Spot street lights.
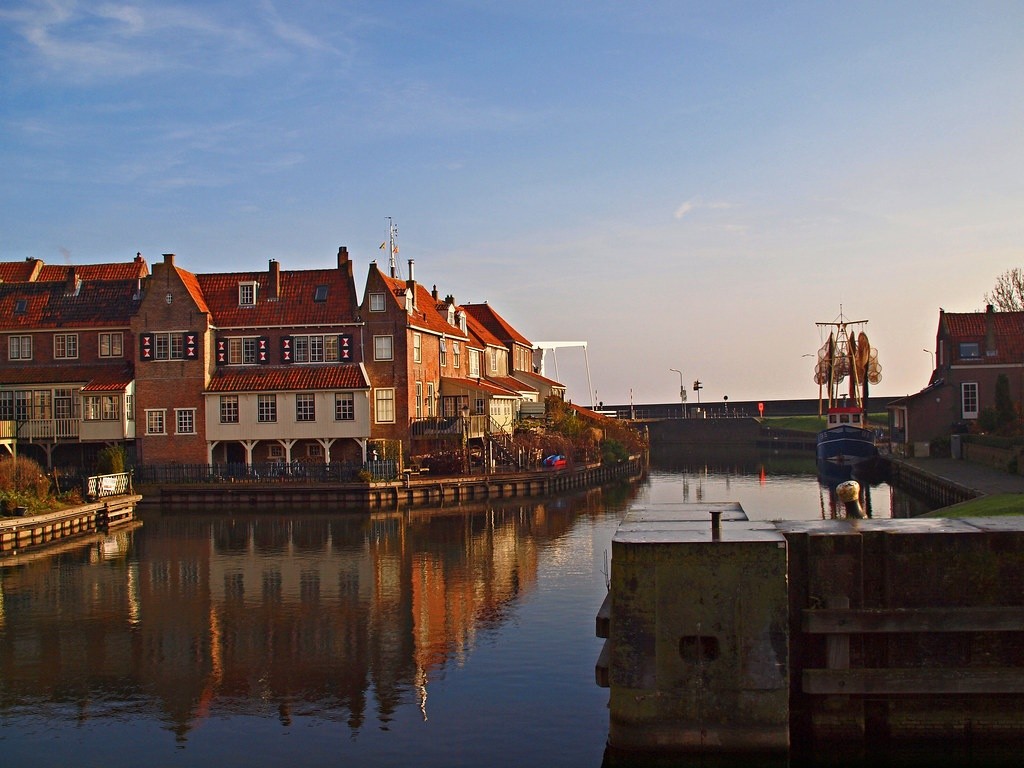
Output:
[924,349,934,370]
[669,369,684,413]
[461,405,473,475]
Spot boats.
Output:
[543,454,566,465]
[813,393,879,482]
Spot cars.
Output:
[192,473,224,484]
